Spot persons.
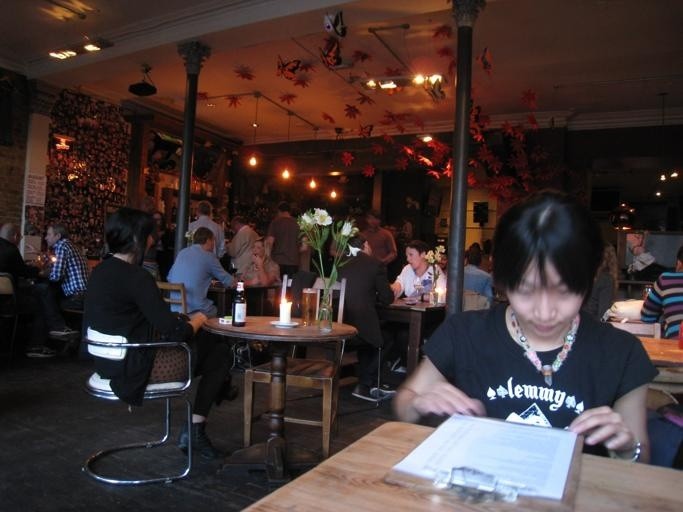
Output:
[166,227,235,368]
[225,216,262,276]
[463,249,494,303]
[80,208,240,459]
[0,222,81,358]
[582,242,619,318]
[640,244,683,339]
[327,228,352,263]
[24,221,89,313]
[265,201,308,317]
[400,216,414,241]
[357,209,397,280]
[332,233,398,403]
[235,238,282,365]
[383,240,446,374]
[391,186,660,464]
[437,252,450,274]
[145,211,172,295]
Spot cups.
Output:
[431,290,439,307]
[301,287,319,326]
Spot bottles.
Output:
[233,281,245,326]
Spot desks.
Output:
[243,423,683,508]
[384,302,446,382]
[202,316,358,485]
[638,336,683,367]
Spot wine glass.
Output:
[411,276,423,304]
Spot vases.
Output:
[314,291,333,330]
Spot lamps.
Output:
[330,137,338,201]
[356,72,445,91]
[281,114,291,180]
[309,127,318,189]
[250,96,259,167]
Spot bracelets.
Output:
[607,432,641,462]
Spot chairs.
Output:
[155,281,187,315]
[607,323,660,338]
[243,275,347,460]
[83,339,192,485]
[1,274,24,341]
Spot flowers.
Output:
[296,207,361,322]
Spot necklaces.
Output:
[510,308,580,386]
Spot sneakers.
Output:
[351,383,382,403]
[26,344,56,358]
[370,384,398,400]
[49,327,80,336]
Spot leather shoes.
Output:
[174,420,230,462]
[215,382,239,406]
